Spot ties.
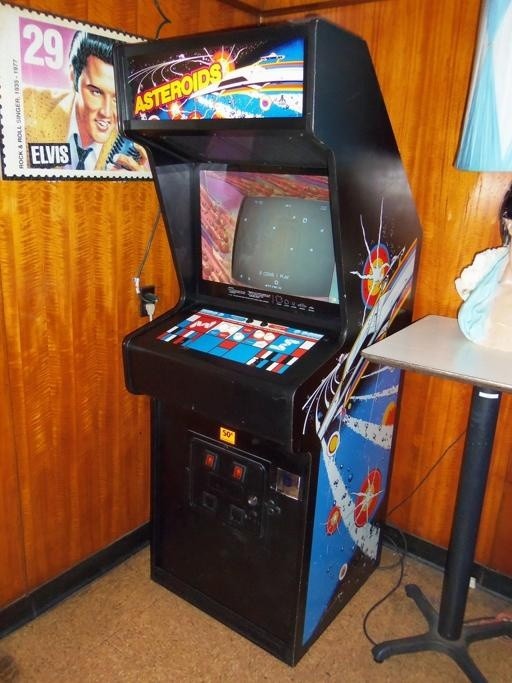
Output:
[73,133,93,170]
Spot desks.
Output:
[357,313,511,683]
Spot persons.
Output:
[19,30,149,175]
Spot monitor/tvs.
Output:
[231,196,339,305]
